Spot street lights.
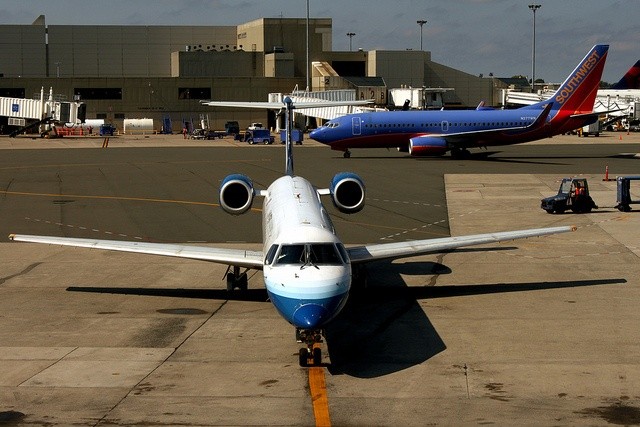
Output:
[417,19,427,50]
[346,32,355,53]
[527,3,542,92]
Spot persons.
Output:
[182,127,188,139]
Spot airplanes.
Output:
[309,43,610,161]
[9,97,579,365]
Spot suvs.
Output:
[248,130,271,144]
[100,123,116,136]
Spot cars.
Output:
[248,122,265,130]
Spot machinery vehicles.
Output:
[542,176,590,213]
